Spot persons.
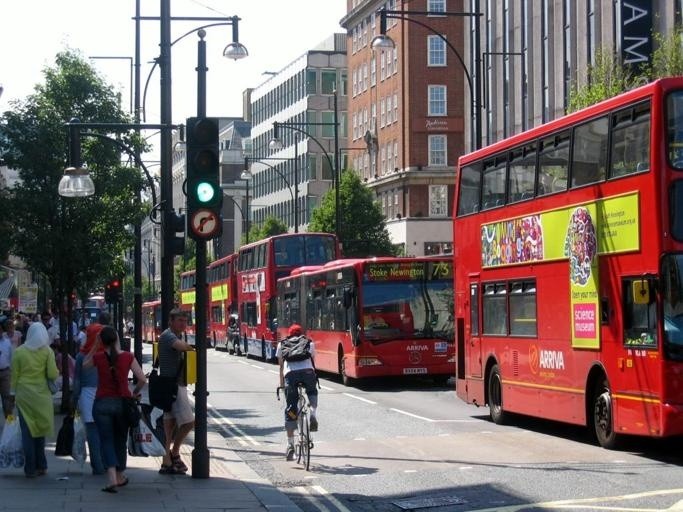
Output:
[82,327,146,495]
[0,311,120,477]
[275,324,318,461]
[158,308,195,475]
[641,283,683,331]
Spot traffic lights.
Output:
[105,278,110,303]
[186,117,220,204]
[112,277,122,302]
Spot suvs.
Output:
[224,312,241,355]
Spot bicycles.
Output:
[276,383,321,470]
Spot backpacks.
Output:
[281,335,312,362]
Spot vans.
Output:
[74,306,103,324]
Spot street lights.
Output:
[370,0,484,151]
[266,89,341,237]
[56,117,221,478]
[239,130,299,233]
[131,0,247,375]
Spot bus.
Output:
[272,255,456,388]
[208,253,238,351]
[234,233,342,364]
[123,300,180,344]
[452,72,682,452]
[175,266,211,348]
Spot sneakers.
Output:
[310,416,317,431]
[286,443,294,461]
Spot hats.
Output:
[289,324,303,337]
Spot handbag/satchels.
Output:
[0,415,25,468]
[128,404,166,456]
[55,410,98,464]
[48,380,58,394]
[149,370,178,412]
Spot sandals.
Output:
[159,455,187,474]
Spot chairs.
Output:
[471,191,542,212]
[637,162,648,171]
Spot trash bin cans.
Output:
[124,338,130,351]
